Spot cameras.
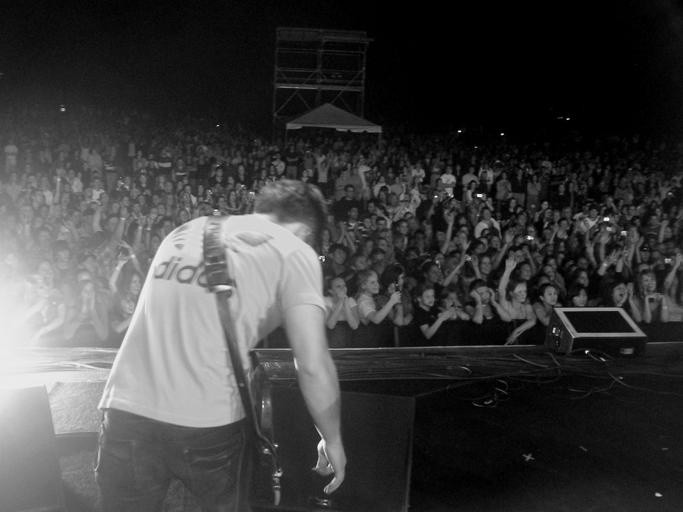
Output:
[621,231,628,236]
[318,255,325,262]
[606,227,612,231]
[524,235,534,241]
[476,194,483,198]
[603,217,610,221]
[664,259,672,263]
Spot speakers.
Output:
[260,390,417,510]
[542,306,649,359]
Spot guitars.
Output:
[250,353,283,512]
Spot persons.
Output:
[1,96,682,347]
[87,176,349,511]
